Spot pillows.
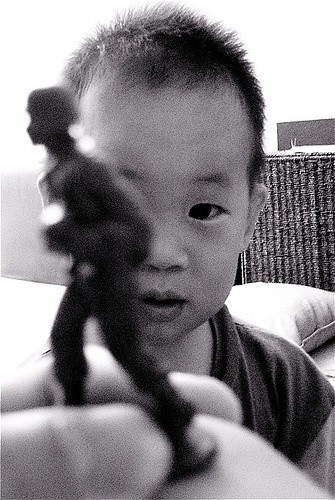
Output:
[224,282,335,353]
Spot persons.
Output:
[0,344,335,500]
[1,1,335,500]
[24,87,217,479]
